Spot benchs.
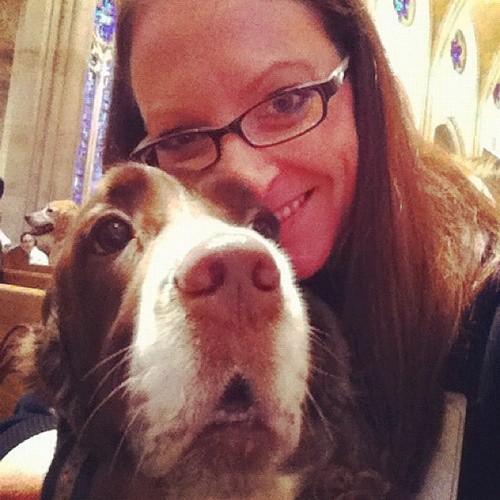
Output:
[0,264,52,420]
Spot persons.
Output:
[0,178,13,256]
[0,0,500,500]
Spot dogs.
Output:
[14,161,390,500]
[23,199,80,265]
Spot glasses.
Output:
[128,53,353,178]
